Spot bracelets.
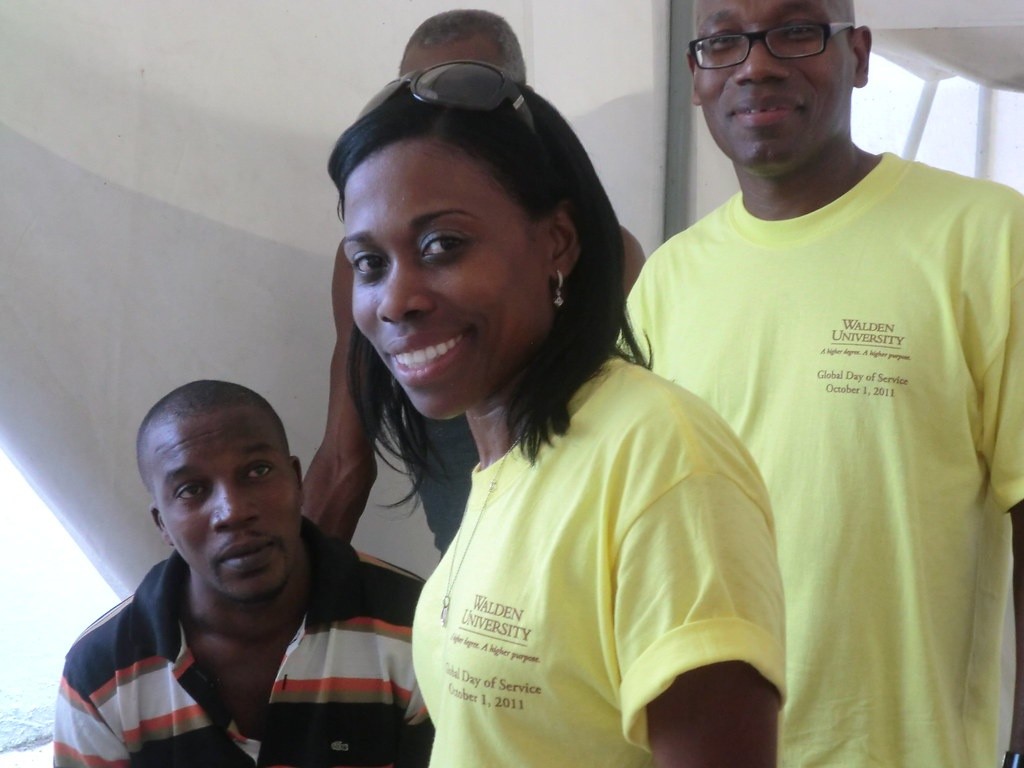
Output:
[1002,750,1024,768]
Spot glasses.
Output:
[359,59,535,134]
[688,23,855,69]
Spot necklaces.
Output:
[440,430,528,628]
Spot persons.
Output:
[299,5,648,566]
[326,67,791,768]
[50,378,436,768]
[610,0,1024,768]
